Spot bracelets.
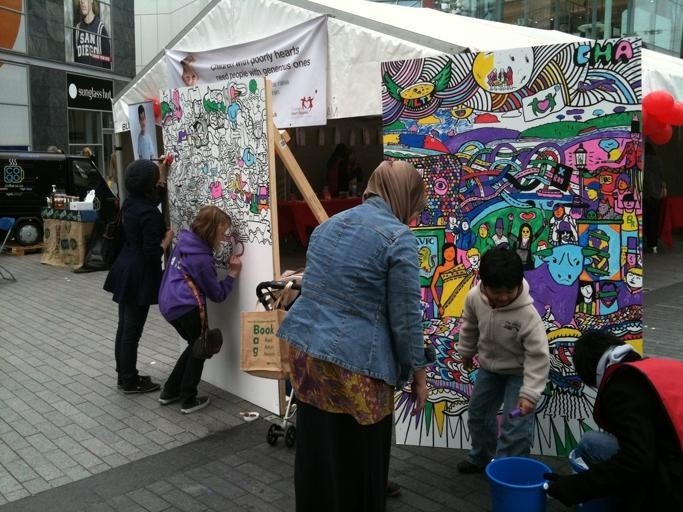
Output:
[229,270,239,277]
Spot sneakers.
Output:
[117,375,160,394]
[457,461,479,474]
[181,396,209,414]
[158,390,183,404]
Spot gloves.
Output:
[543,473,596,507]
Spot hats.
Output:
[124,159,159,194]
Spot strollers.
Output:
[256,281,301,448]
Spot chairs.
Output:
[0,217,17,281]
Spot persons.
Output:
[543,326,683,512]
[178,54,201,88]
[457,244,551,477]
[102,154,175,395]
[156,205,242,416]
[274,160,437,510]
[72,0,111,69]
[135,105,157,164]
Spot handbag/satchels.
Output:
[101,223,125,264]
[193,328,223,359]
[239,309,291,380]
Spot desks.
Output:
[39,220,94,271]
[276,196,360,247]
[640,196,682,253]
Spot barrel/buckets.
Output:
[486,455,555,511]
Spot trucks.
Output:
[0,151,120,246]
